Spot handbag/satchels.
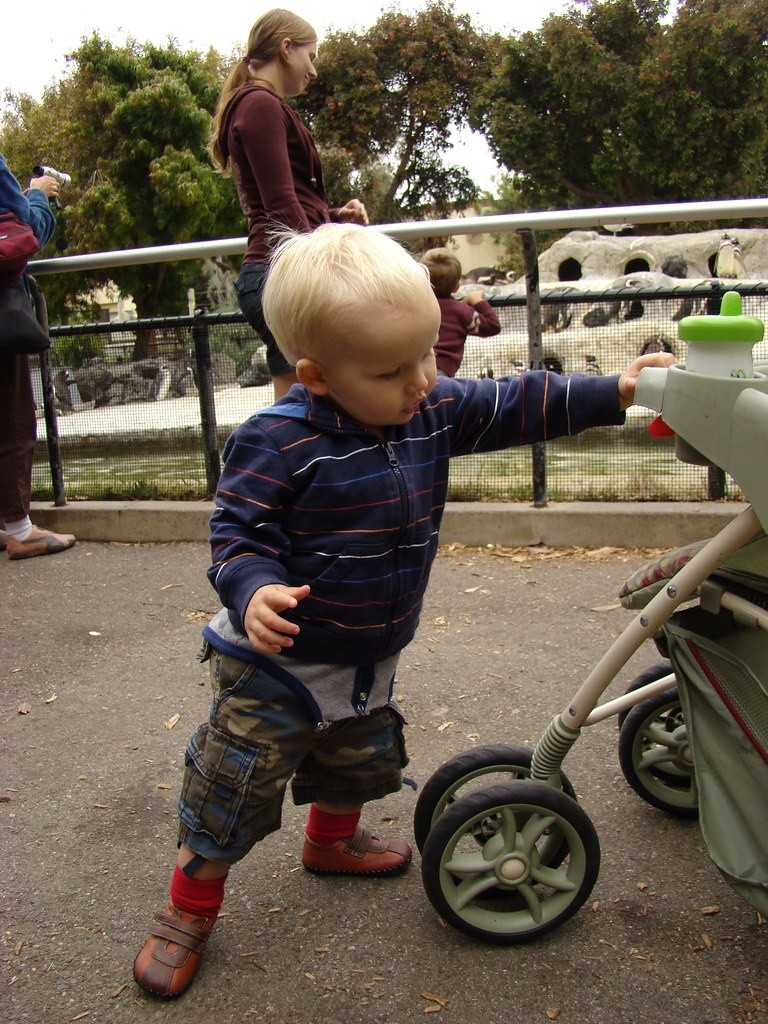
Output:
[0,211,39,281]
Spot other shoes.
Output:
[0,524,76,560]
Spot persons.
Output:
[0,151,76,559]
[418,248,501,378]
[209,9,369,403]
[132,222,677,1000]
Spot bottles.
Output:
[676,291,764,379]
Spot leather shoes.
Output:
[302,823,412,874]
[133,898,218,997]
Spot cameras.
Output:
[32,167,72,189]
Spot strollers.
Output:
[411,291,768,947]
